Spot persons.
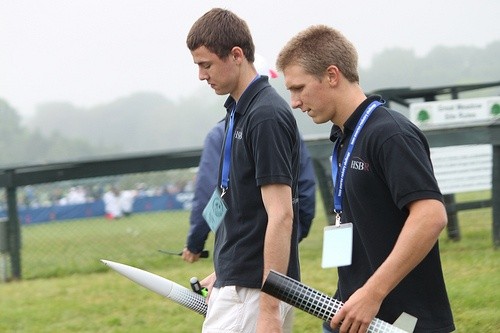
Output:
[277,26,456,333]
[181,113,317,263]
[187,8,302,333]
